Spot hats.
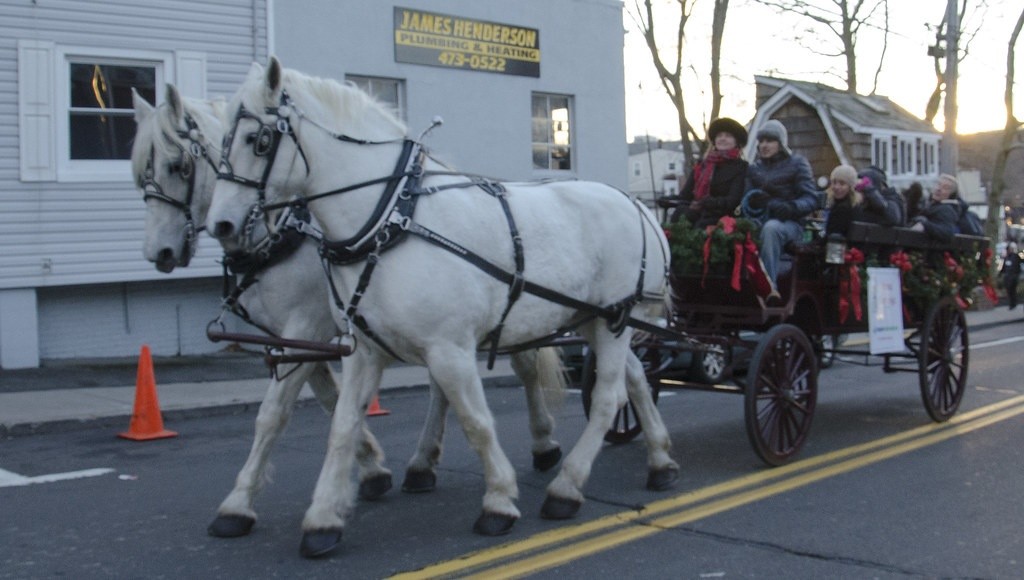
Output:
[755,119,793,158]
[857,166,887,191]
[830,165,857,208]
[708,118,748,149]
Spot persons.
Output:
[744,119,821,307]
[671,118,749,229]
[819,166,962,236]
[997,244,1022,310]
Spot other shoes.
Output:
[766,291,783,306]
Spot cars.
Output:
[558,323,849,389]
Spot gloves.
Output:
[775,202,793,218]
[749,191,771,208]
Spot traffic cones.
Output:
[114,346,180,441]
[365,392,390,417]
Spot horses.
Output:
[205,55,678,558]
[131,83,565,538]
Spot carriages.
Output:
[131,54,993,559]
